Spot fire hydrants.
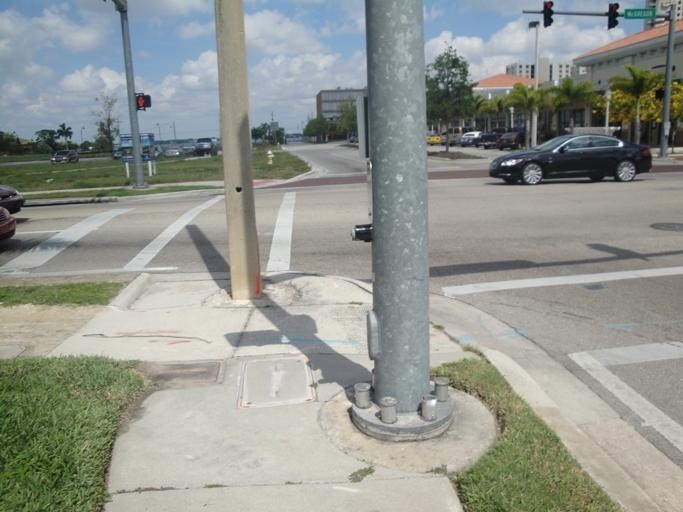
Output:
[267,150,274,165]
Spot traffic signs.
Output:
[624,8,655,19]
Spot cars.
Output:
[115,151,122,158]
[489,134,652,185]
[164,149,183,157]
[427,127,524,151]
[0,183,25,241]
[51,150,79,164]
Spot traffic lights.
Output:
[609,3,619,28]
[544,1,554,26]
[136,95,151,107]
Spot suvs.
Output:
[196,138,217,157]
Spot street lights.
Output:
[528,21,538,146]
